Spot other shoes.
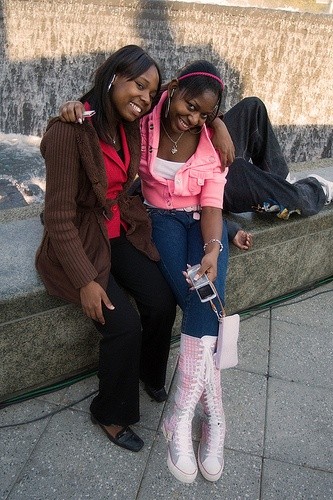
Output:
[307,174,333,206]
[276,209,301,221]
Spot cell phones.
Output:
[187,264,216,303]
[82,111,96,117]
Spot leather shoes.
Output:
[89,415,142,452]
[143,381,166,402]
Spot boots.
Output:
[160,332,210,483]
[197,334,226,481]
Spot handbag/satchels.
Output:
[215,314,240,369]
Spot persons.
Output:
[58,60,229,483]
[35,45,236,452]
[222,97,333,249]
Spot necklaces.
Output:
[161,121,184,154]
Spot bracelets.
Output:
[204,239,223,252]
